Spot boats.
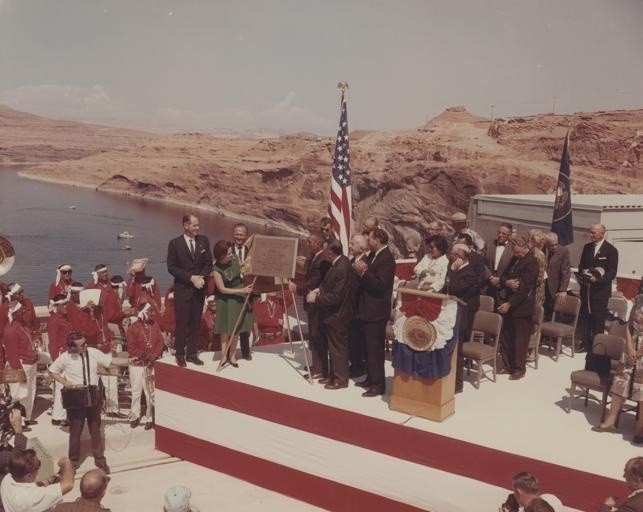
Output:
[117,230,134,239]
[69,205,75,209]
[119,245,130,251]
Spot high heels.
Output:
[229,359,238,368]
[219,358,227,367]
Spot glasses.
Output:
[75,341,88,348]
[61,270,73,275]
[15,288,24,295]
[33,460,41,471]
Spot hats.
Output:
[80,469,111,500]
[451,212,472,223]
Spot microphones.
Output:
[445,276,451,296]
[411,273,430,279]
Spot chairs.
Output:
[36,351,54,403]
[111,334,133,395]
[385,278,642,435]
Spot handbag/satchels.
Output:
[585,343,611,375]
[606,374,633,400]
[399,269,421,291]
[512,279,540,297]
[0,360,27,384]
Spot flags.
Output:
[551,127,575,247]
[327,89,353,258]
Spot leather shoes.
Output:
[52,420,69,425]
[348,361,385,396]
[631,436,642,445]
[186,357,203,366]
[509,371,525,380]
[21,425,31,431]
[574,343,584,353]
[493,366,512,375]
[98,463,110,474]
[245,354,252,360]
[175,358,186,367]
[592,423,616,434]
[303,366,347,389]
[108,412,152,429]
[25,420,37,425]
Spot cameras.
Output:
[501,493,519,512]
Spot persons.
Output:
[1,264,165,511]
[592,277,643,443]
[290,215,570,397]
[502,456,643,511]
[163,216,296,367]
[575,224,618,352]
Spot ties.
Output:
[237,245,242,264]
[190,241,195,260]
[547,251,551,261]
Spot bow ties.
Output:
[498,242,506,247]
